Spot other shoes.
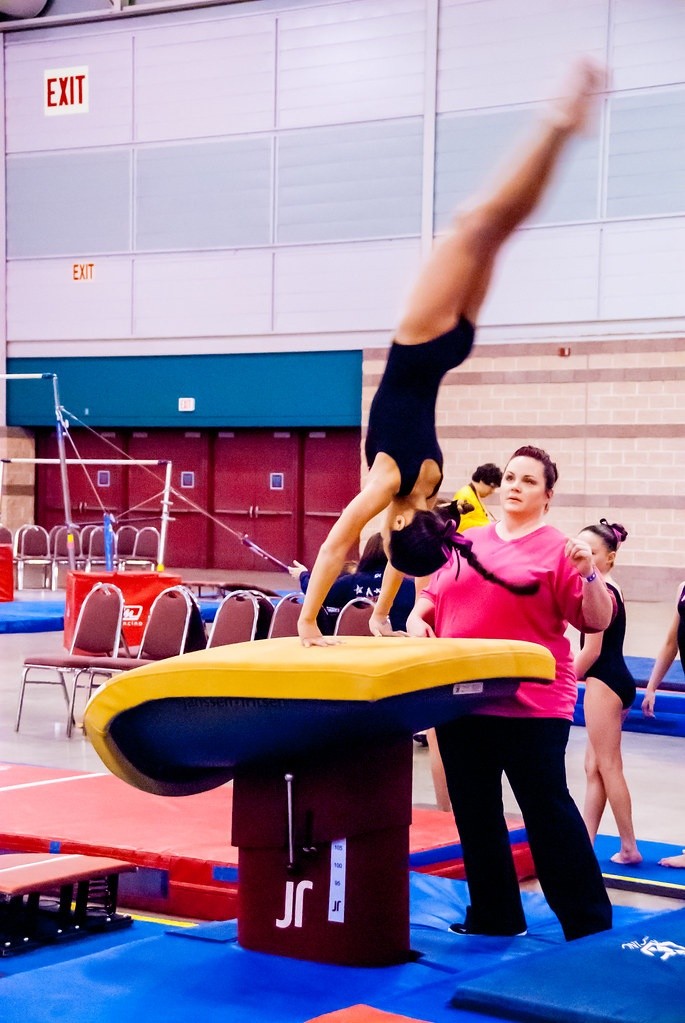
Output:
[448,923,530,940]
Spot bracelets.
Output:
[586,564,596,582]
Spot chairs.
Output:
[0,523,160,592]
[172,583,378,653]
[65,588,195,740]
[17,583,123,738]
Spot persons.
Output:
[404,444,615,937]
[297,43,605,648]
[287,532,416,634]
[641,579,685,871]
[575,519,644,865]
[453,463,503,537]
